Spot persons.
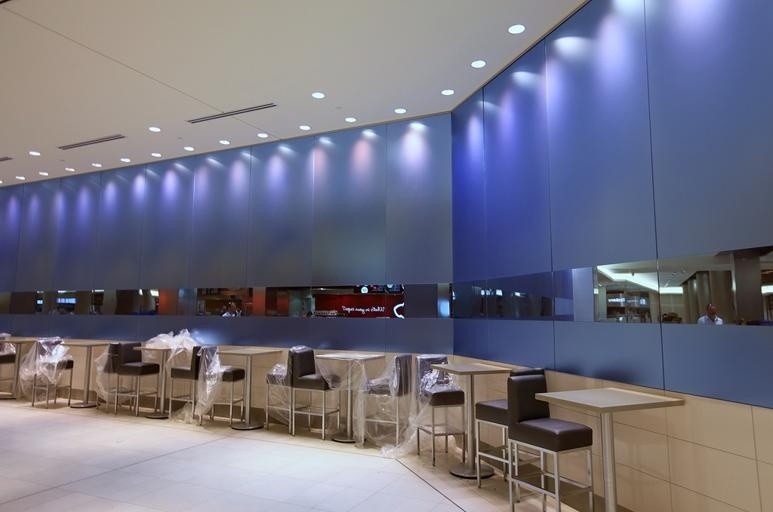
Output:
[695,301,721,326]
[221,301,237,318]
